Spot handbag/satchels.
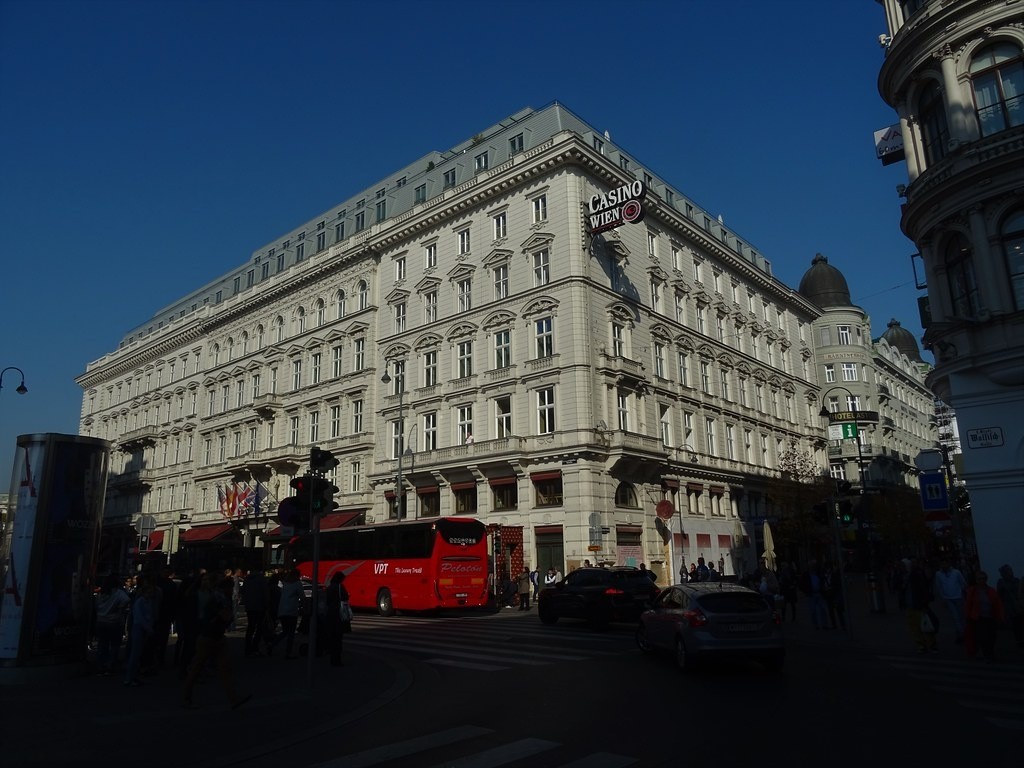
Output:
[339,601,353,622]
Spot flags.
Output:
[218,476,269,519]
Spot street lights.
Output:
[820,387,874,554]
[676,444,699,583]
[381,358,402,521]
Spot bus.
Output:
[285,517,488,618]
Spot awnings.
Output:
[178,526,234,543]
[134,531,163,555]
[259,511,363,540]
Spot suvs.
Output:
[538,565,662,625]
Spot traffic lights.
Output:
[812,505,832,528]
[291,478,311,535]
[840,510,854,523]
[314,481,325,512]
[326,484,339,514]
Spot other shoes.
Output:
[525,606,530,611]
[285,654,299,660]
[228,694,252,710]
[506,606,511,609]
[183,702,199,710]
[96,670,112,678]
[122,679,139,687]
[518,607,524,611]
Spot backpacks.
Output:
[531,571,538,582]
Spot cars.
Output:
[636,582,784,659]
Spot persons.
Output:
[688,558,724,582]
[496,565,564,611]
[799,559,848,632]
[955,565,1024,663]
[599,563,604,569]
[738,560,798,624]
[93,559,352,711]
[886,556,941,654]
[640,563,657,582]
[583,560,589,568]
